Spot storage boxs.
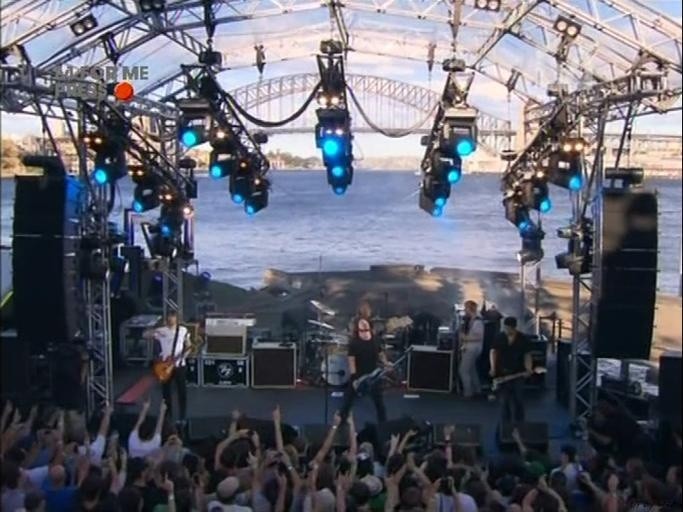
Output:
[187,354,250,388]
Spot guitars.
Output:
[151,336,204,384]
[352,346,413,398]
[481,365,548,402]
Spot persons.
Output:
[488,316,534,439]
[480,299,506,333]
[0,395,683,512]
[346,302,384,398]
[339,318,396,422]
[457,300,485,402]
[142,310,192,418]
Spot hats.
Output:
[216,475,239,499]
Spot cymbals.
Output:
[370,317,386,322]
[310,299,336,317]
[308,319,335,330]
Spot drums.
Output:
[326,334,351,357]
[321,351,350,389]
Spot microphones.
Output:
[331,370,345,376]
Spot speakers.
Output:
[200,356,250,389]
[406,344,453,394]
[0,174,92,411]
[110,412,549,458]
[555,186,683,466]
[183,356,201,388]
[251,342,297,389]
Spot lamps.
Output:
[501,104,592,273]
[417,109,480,219]
[91,80,270,237]
[316,109,354,196]
